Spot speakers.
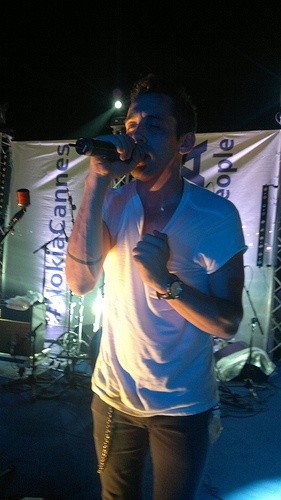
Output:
[0,302,47,356]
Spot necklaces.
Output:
[159,177,184,216]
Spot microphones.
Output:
[60,224,69,242]
[9,188,30,226]
[68,136,147,161]
[10,343,16,356]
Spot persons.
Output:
[64,79,246,500]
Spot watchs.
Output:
[155,274,184,300]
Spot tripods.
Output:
[228,287,270,403]
[2,290,91,402]
